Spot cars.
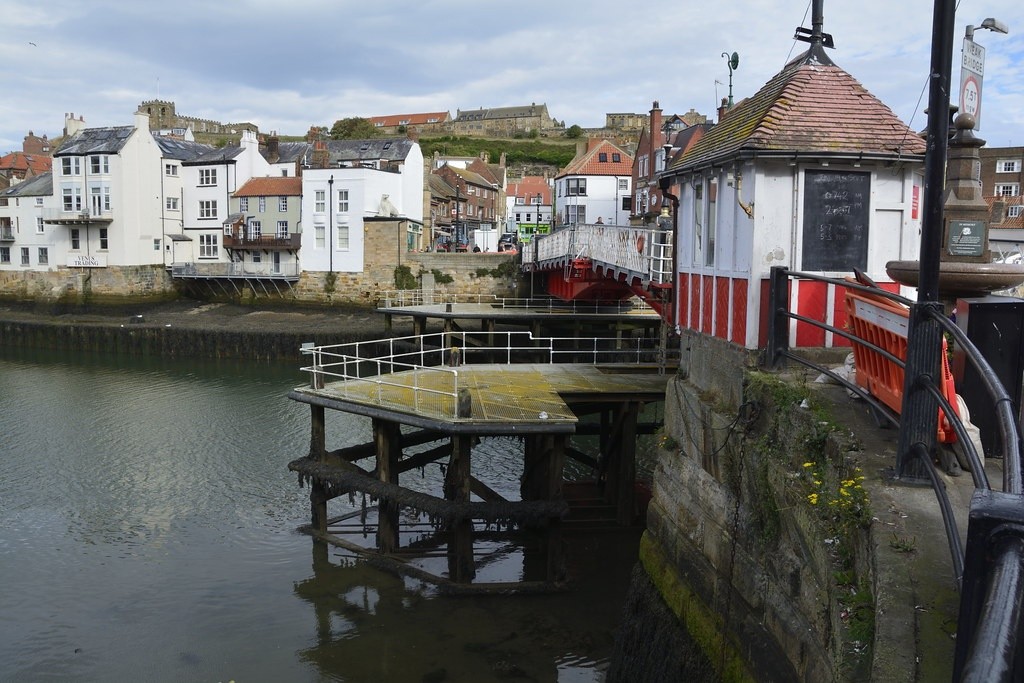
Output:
[502,234,516,245]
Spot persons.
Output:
[447,239,451,251]
[595,217,604,224]
[473,244,481,253]
